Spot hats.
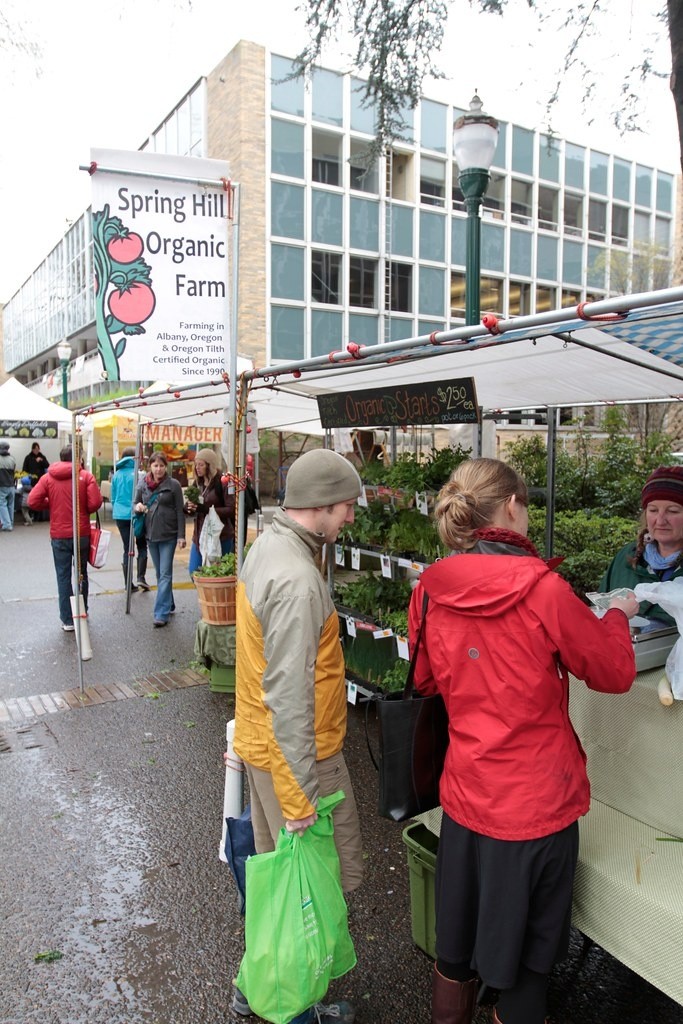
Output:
[0,440,9,450]
[282,447,362,510]
[640,466,683,511]
[194,448,218,474]
[22,474,32,485]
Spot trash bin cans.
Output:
[401,803,440,959]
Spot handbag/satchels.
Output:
[224,802,259,919]
[364,590,449,821]
[199,505,225,568]
[234,790,358,1024]
[88,510,112,568]
[132,490,159,537]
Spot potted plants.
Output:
[190,554,237,626]
[346,440,471,702]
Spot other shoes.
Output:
[171,604,175,611]
[311,1001,356,1023]
[0,526,14,531]
[61,620,74,632]
[24,520,33,526]
[123,564,138,592]
[232,988,252,1016]
[153,619,168,626]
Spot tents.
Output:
[0,375,222,474]
[71,284,683,694]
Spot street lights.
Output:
[57,335,72,409]
[452,87,499,326]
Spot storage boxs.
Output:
[402,805,446,961]
[193,620,237,694]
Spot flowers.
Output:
[13,469,38,480]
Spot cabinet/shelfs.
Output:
[326,538,432,703]
[564,666,683,1009]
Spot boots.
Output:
[136,557,149,590]
[432,960,547,1024]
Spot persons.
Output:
[183,448,235,587]
[132,450,187,627]
[598,465,683,627]
[231,448,365,1024]
[0,441,33,531]
[409,458,640,1024]
[110,445,152,593]
[23,443,49,488]
[28,444,103,632]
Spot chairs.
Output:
[98,480,111,522]
